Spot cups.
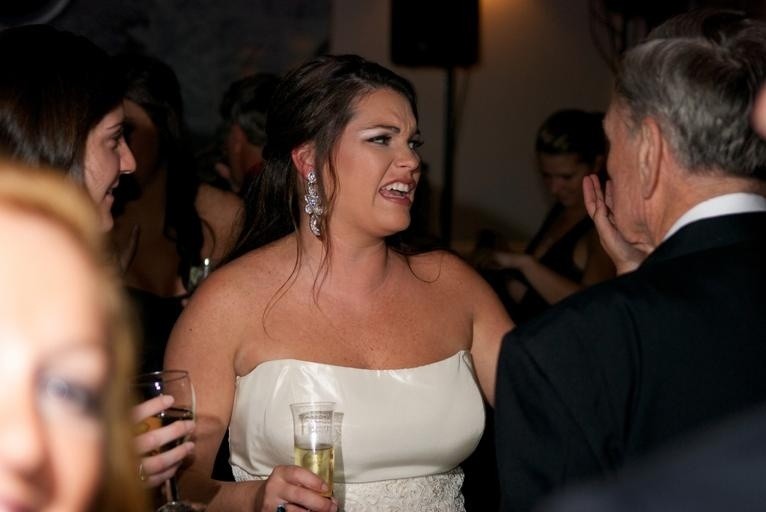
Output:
[286,400,335,500]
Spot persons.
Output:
[465,39,766,511]
[162,54,516,512]
[1,158,154,512]
[3,22,197,489]
[106,52,612,400]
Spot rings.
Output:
[136,463,145,480]
[277,502,289,512]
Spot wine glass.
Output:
[126,369,208,511]
